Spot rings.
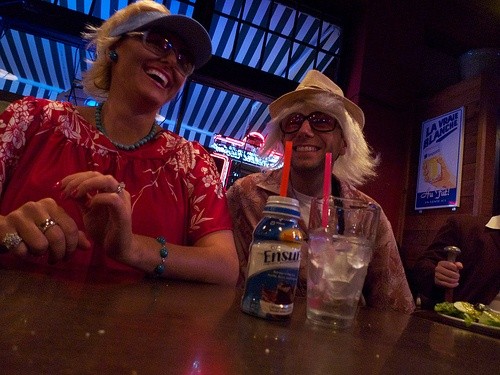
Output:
[38,217,56,233]
[116,182,125,193]
[2,232,23,251]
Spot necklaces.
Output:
[95,101,157,149]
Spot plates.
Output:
[437,312,500,334]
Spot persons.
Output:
[224,70,500,311]
[0,0,240,285]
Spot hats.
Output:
[269,70,366,131]
[108,10,213,71]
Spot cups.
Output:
[424,143,442,183]
[304,196,382,330]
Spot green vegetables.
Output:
[435,301,500,327]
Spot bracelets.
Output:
[146,236,168,278]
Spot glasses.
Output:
[125,30,195,77]
[279,111,338,134]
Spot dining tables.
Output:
[0,264,500,375]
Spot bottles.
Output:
[241,194,304,325]
